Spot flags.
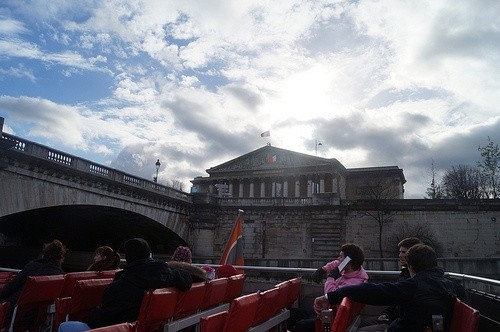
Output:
[262,131,271,137]
[269,153,280,162]
[218,215,245,275]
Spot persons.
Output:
[201,266,216,280]
[216,264,237,278]
[313,244,463,332]
[87,246,121,270]
[297,244,369,332]
[166,246,208,283]
[103,238,192,325]
[0,240,68,331]
[394,237,425,281]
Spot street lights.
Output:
[315,138,322,193]
[154,159,160,183]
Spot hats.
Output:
[199,265,216,280]
[171,246,192,265]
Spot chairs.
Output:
[0,269,480,332]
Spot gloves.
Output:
[328,267,341,281]
[311,267,325,285]
